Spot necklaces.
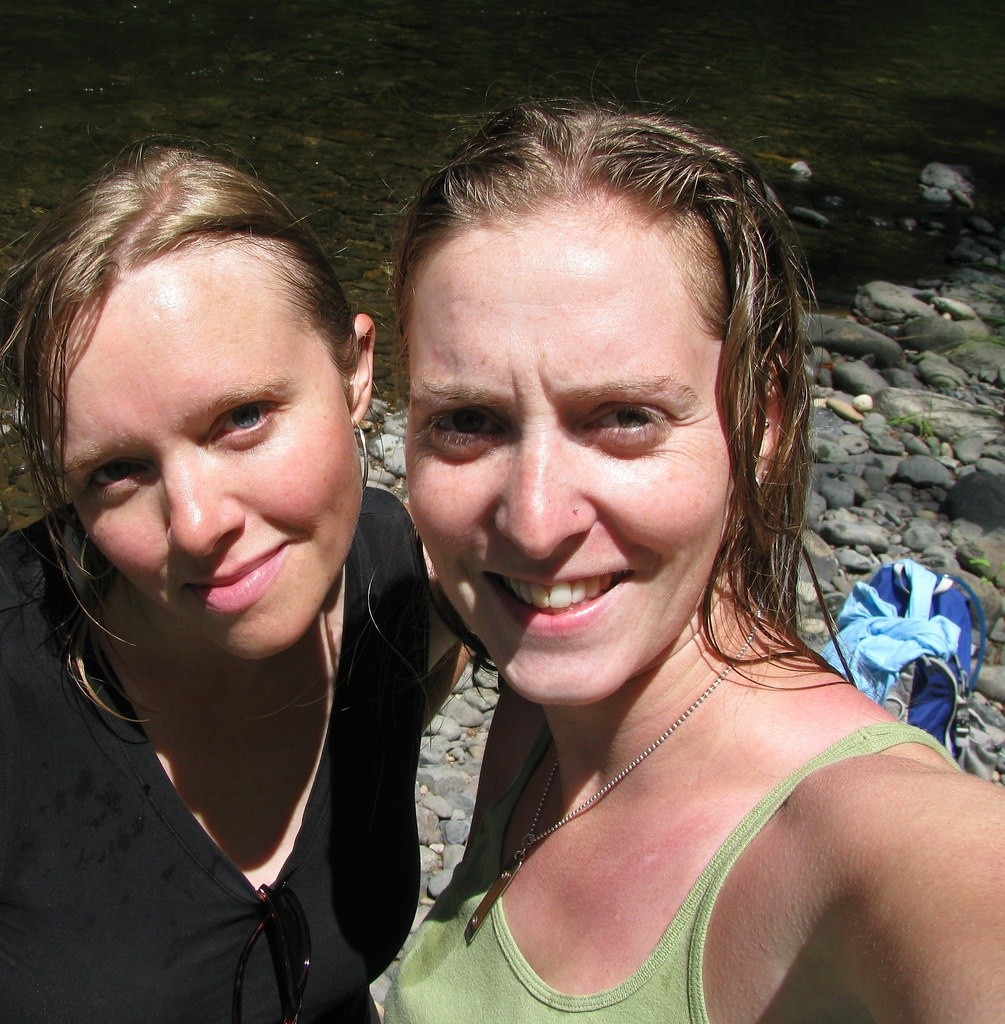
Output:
[463,608,762,946]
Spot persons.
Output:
[389,97,1005,1024]
[0,143,469,1024]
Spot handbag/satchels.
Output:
[819,561,987,761]
